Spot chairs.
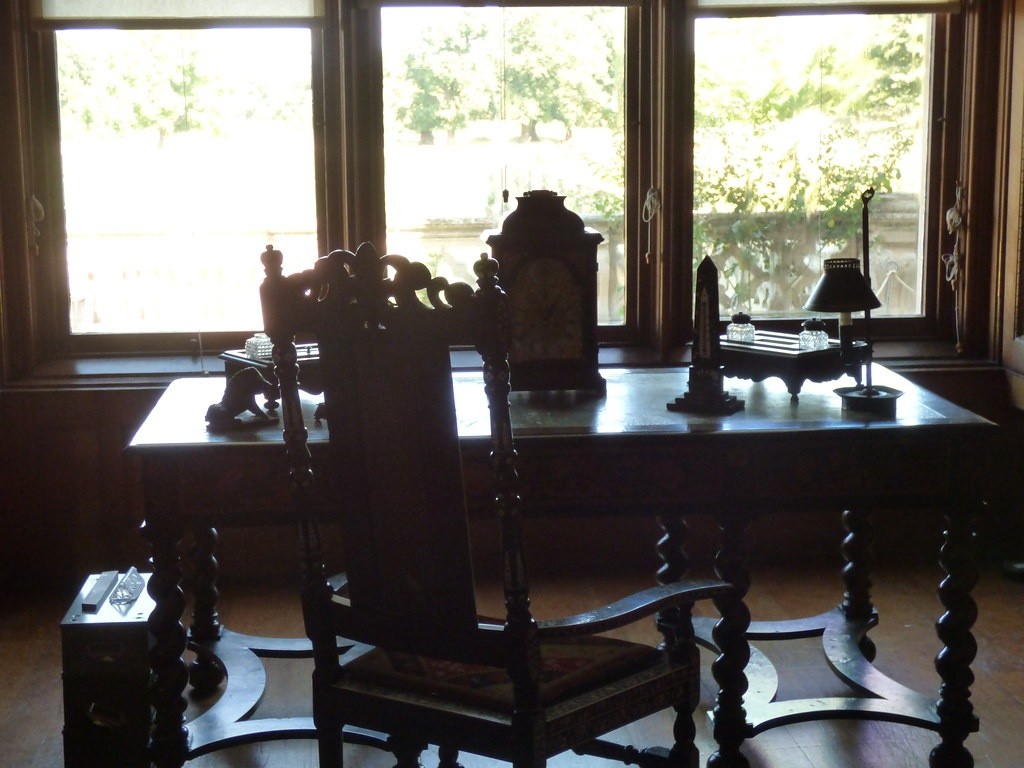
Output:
[259,242,752,768]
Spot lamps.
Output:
[802,257,882,348]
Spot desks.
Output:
[124,361,996,768]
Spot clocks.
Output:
[504,255,584,361]
